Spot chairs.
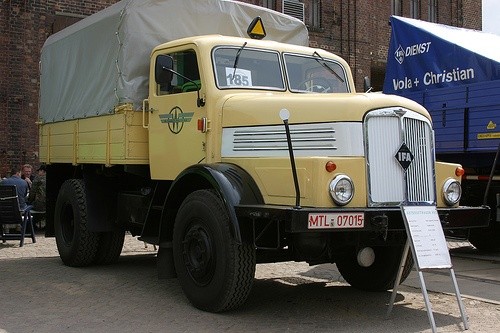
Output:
[0,184,46,247]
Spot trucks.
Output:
[36,1,491,314]
[383,14,500,253]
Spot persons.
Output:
[0,166,33,232]
[12,164,35,205]
[26,165,47,233]
